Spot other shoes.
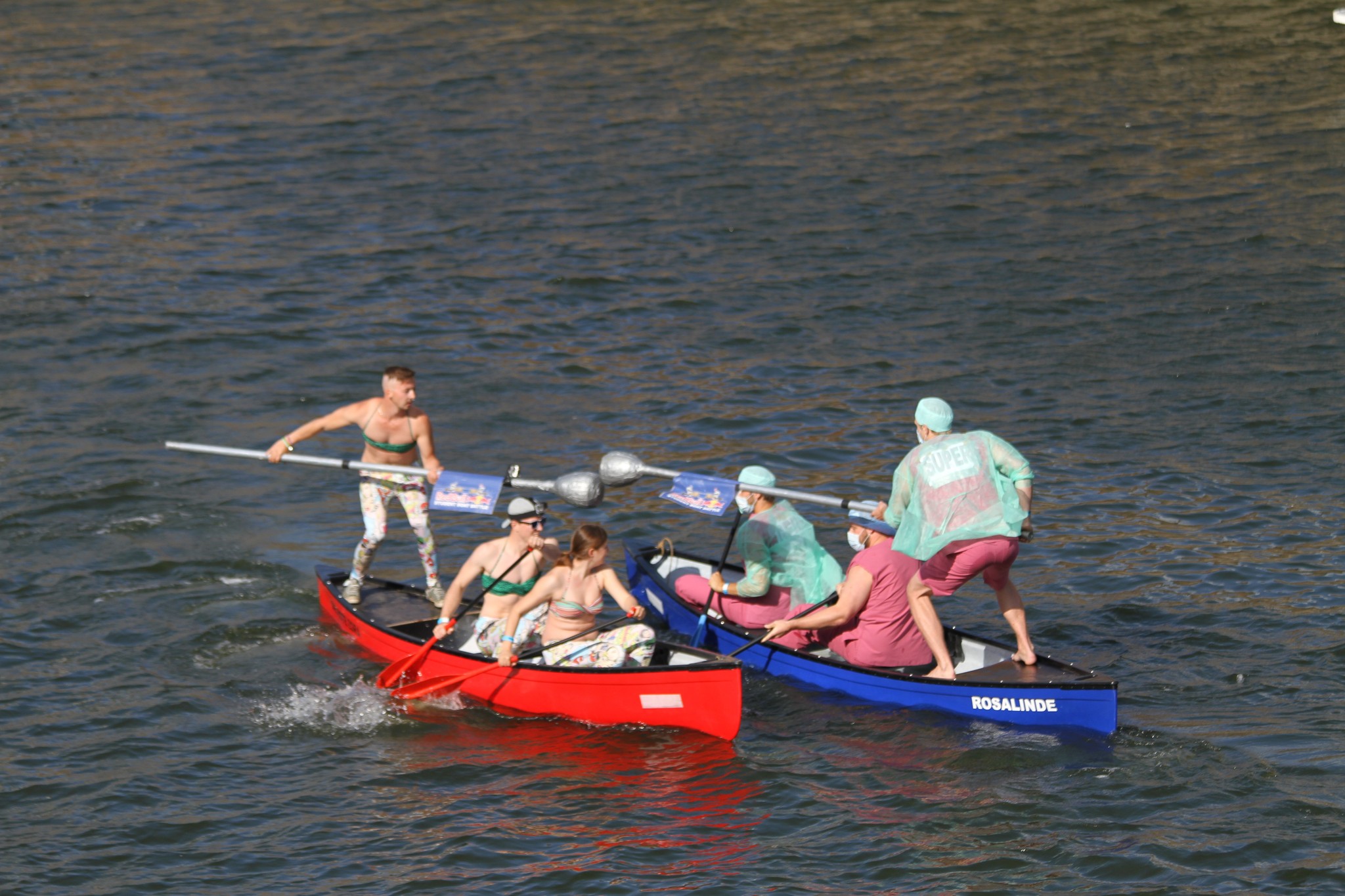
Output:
[342,577,361,604]
[426,581,446,608]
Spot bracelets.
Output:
[502,636,515,643]
[722,581,730,595]
[437,617,450,623]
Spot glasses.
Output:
[519,517,546,528]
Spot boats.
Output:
[313,562,744,741]
[623,534,1118,736]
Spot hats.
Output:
[501,496,543,529]
[915,397,953,433]
[738,465,776,488]
[847,500,896,536]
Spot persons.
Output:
[680,467,844,629]
[872,395,1037,684]
[498,526,656,669]
[262,365,448,608]
[763,501,940,675]
[433,497,559,665]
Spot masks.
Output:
[847,527,870,552]
[735,493,757,515]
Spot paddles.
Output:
[377,544,534,690]
[390,607,637,700]
[686,506,742,648]
[728,593,838,657]
